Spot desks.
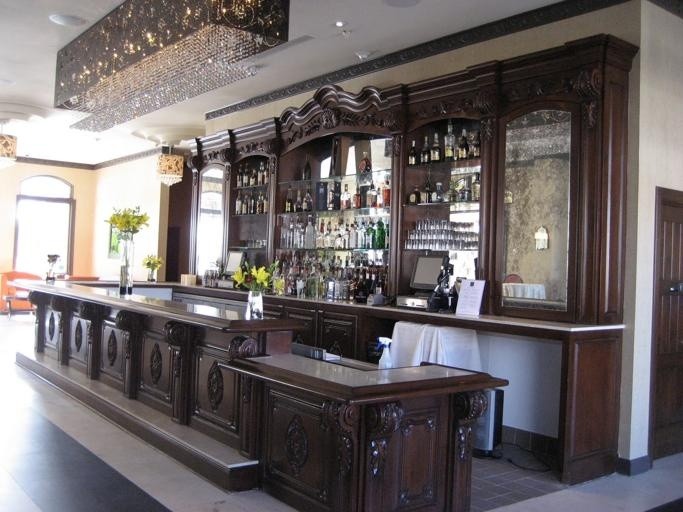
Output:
[216,351,510,512]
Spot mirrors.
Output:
[188,130,233,282]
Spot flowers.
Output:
[100,205,150,264]
[140,254,164,272]
[229,255,287,297]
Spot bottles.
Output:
[233,161,269,216]
[419,136,430,164]
[458,129,469,159]
[279,175,390,250]
[407,172,481,203]
[357,152,370,173]
[470,130,481,157]
[430,132,441,162]
[271,254,389,302]
[408,138,416,164]
[301,152,312,179]
[444,119,455,161]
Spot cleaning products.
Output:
[375,336,393,370]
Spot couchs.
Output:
[0,271,99,319]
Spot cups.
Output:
[246,239,266,248]
[503,283,545,300]
[404,216,478,251]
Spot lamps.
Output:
[51,0,292,137]
[130,108,207,188]
[0,118,18,161]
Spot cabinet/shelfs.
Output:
[264,302,394,365]
[485,33,641,327]
[261,83,404,306]
[220,115,281,287]
[389,61,502,316]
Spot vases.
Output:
[240,290,265,321]
[145,269,157,283]
[114,238,134,296]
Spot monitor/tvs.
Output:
[410,255,449,296]
[224,250,247,280]
